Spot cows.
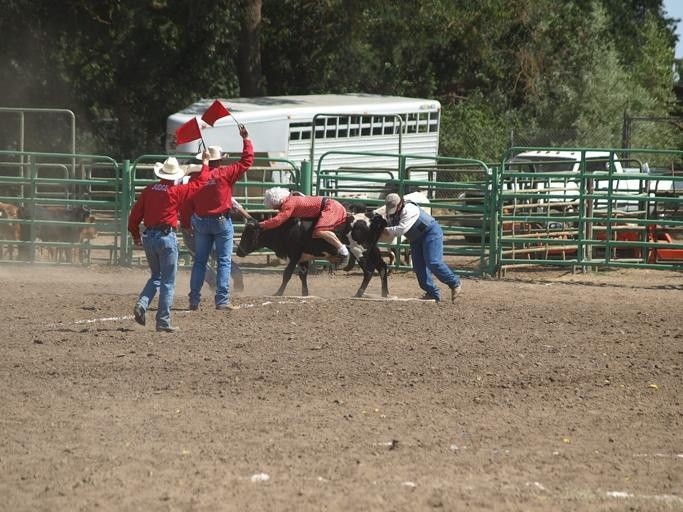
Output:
[0,201,98,268]
[236,210,389,299]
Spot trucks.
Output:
[164,91,443,271]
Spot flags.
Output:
[176,118,201,146]
[200,100,230,126]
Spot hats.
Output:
[196,146,229,161]
[185,164,202,176]
[385,193,401,214]
[154,157,187,180]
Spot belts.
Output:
[321,197,327,210]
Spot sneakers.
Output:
[452,280,461,299]
[420,294,434,299]
[216,303,240,310]
[157,326,179,332]
[190,305,198,310]
[335,253,350,269]
[134,304,145,325]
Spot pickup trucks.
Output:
[454,150,683,242]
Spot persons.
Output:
[128,147,210,332]
[181,163,258,291]
[369,193,462,302]
[181,123,254,311]
[255,186,351,269]
